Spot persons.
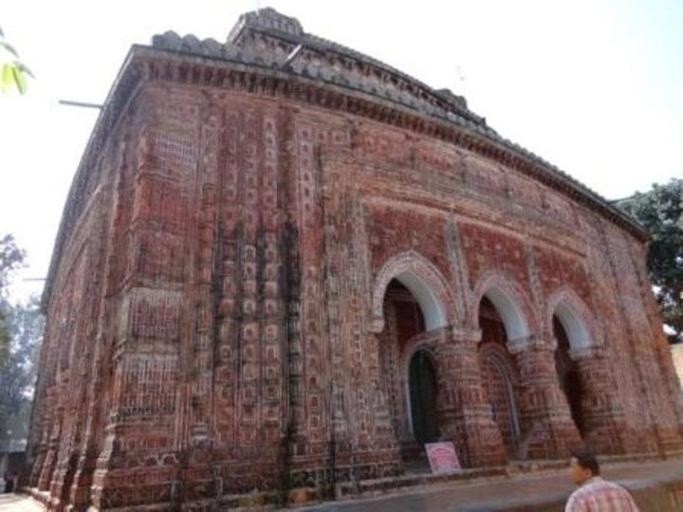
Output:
[2,471,15,493]
[563,449,641,512]
